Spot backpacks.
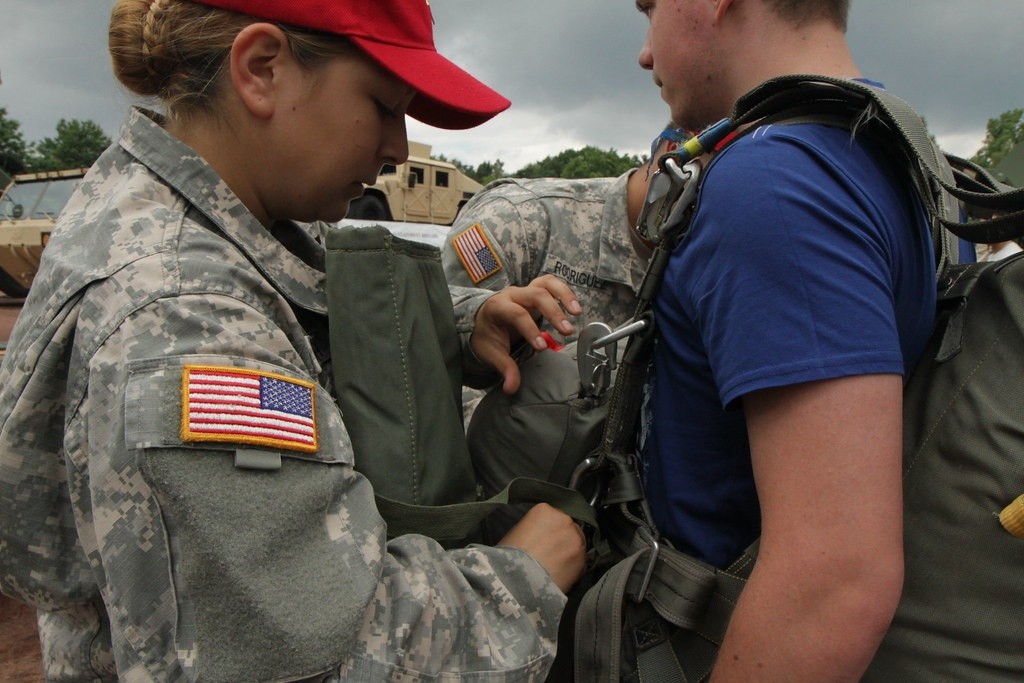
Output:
[574,73,1024,683]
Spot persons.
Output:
[0,0,588,682]
[559,0,972,681]
[438,119,695,500]
[964,179,1022,261]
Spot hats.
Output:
[196,0,511,130]
